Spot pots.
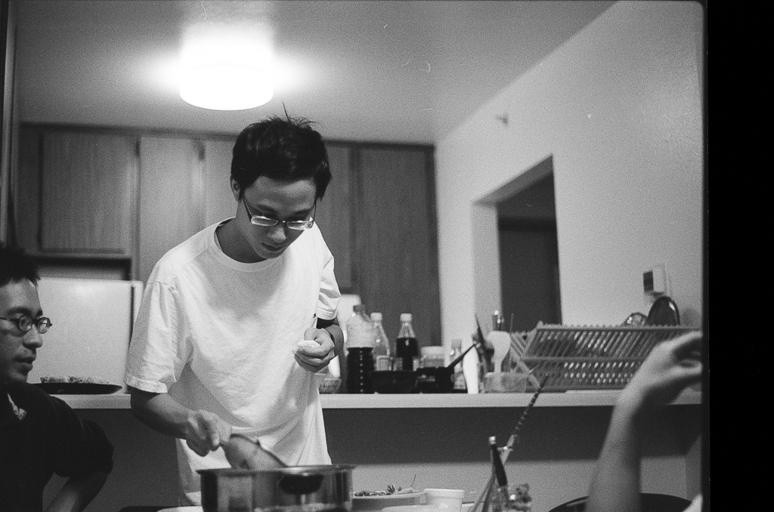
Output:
[193,462,356,511]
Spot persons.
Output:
[0,242,114,512]
[584,330,704,512]
[124,103,345,507]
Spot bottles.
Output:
[447,337,469,394]
[345,304,417,392]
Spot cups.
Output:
[420,345,445,369]
[423,486,465,512]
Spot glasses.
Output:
[0,313,53,334]
[240,186,317,231]
